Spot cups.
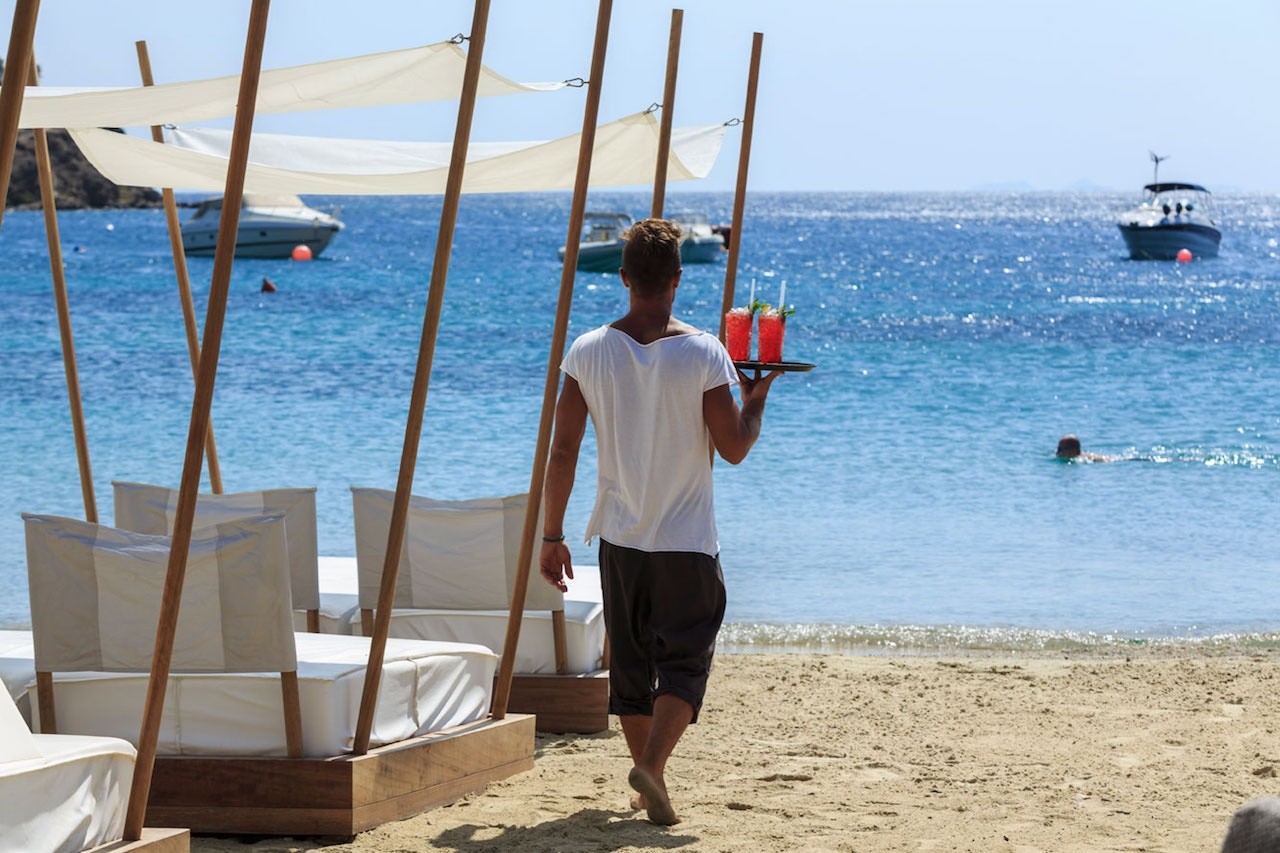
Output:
[724,313,753,360]
[757,315,787,362]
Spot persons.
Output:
[1056,434,1106,463]
[541,217,784,827]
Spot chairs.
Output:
[18,512,539,837]
[351,483,615,739]
[2,682,191,853]
[115,480,360,636]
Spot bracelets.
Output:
[541,534,565,542]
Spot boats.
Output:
[179,189,345,260]
[555,207,725,275]
[1113,169,1223,262]
